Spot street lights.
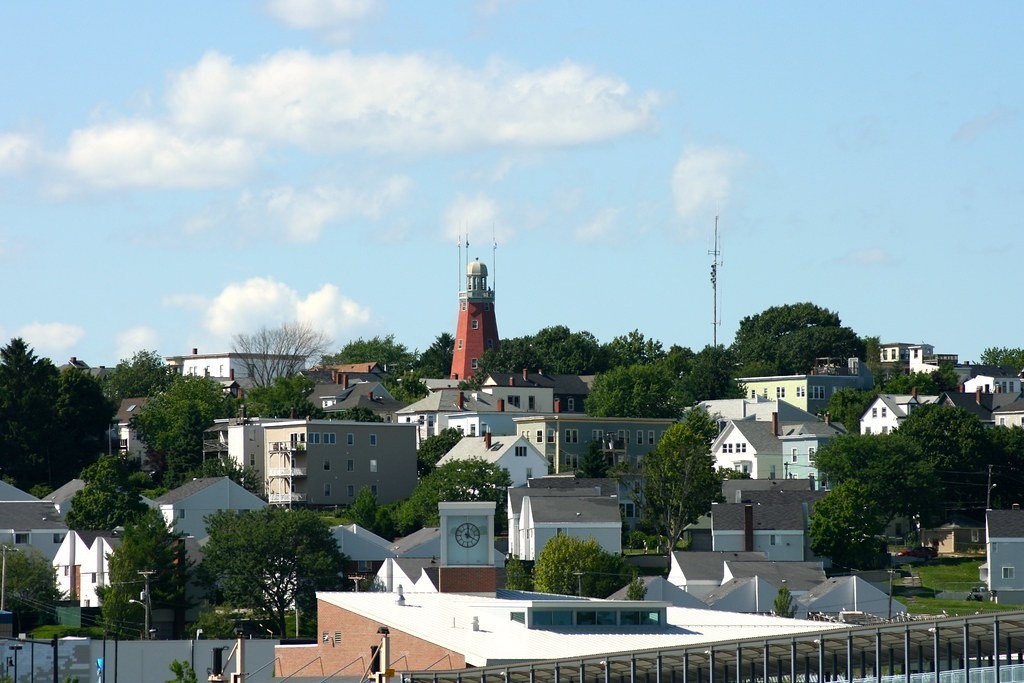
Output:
[987,484,997,509]
[129,599,149,640]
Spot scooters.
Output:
[966,590,983,602]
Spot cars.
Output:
[895,546,938,560]
